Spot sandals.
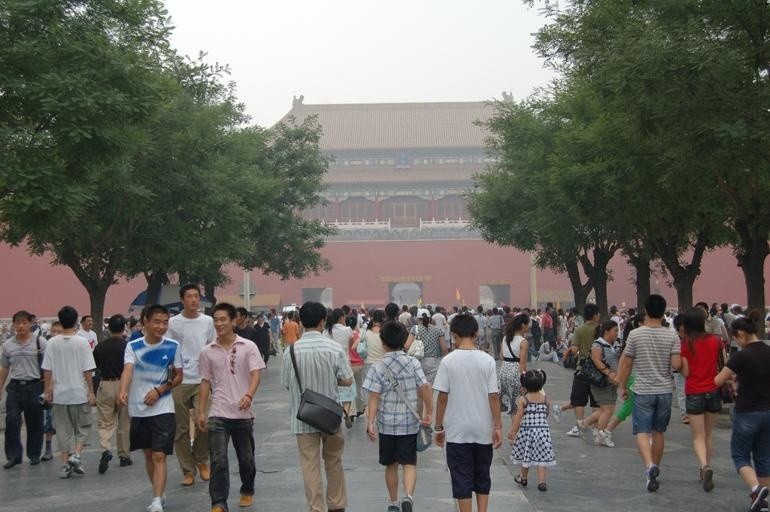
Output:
[538,483,547,491]
[515,475,527,486]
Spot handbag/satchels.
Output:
[721,383,736,403]
[296,389,344,435]
[357,340,367,359]
[416,425,433,452]
[408,339,424,359]
[40,381,52,410]
[581,361,610,387]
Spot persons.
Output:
[0,285,271,511]
[681,308,727,491]
[432,315,503,512]
[268,301,770,447]
[282,301,353,512]
[617,294,681,491]
[508,369,557,490]
[362,322,434,512]
[714,316,770,512]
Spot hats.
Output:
[417,308,431,318]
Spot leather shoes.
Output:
[4,451,253,512]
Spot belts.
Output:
[10,378,39,385]
[100,377,120,381]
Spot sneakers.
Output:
[388,500,401,512]
[402,494,413,512]
[553,404,615,448]
[645,416,769,512]
